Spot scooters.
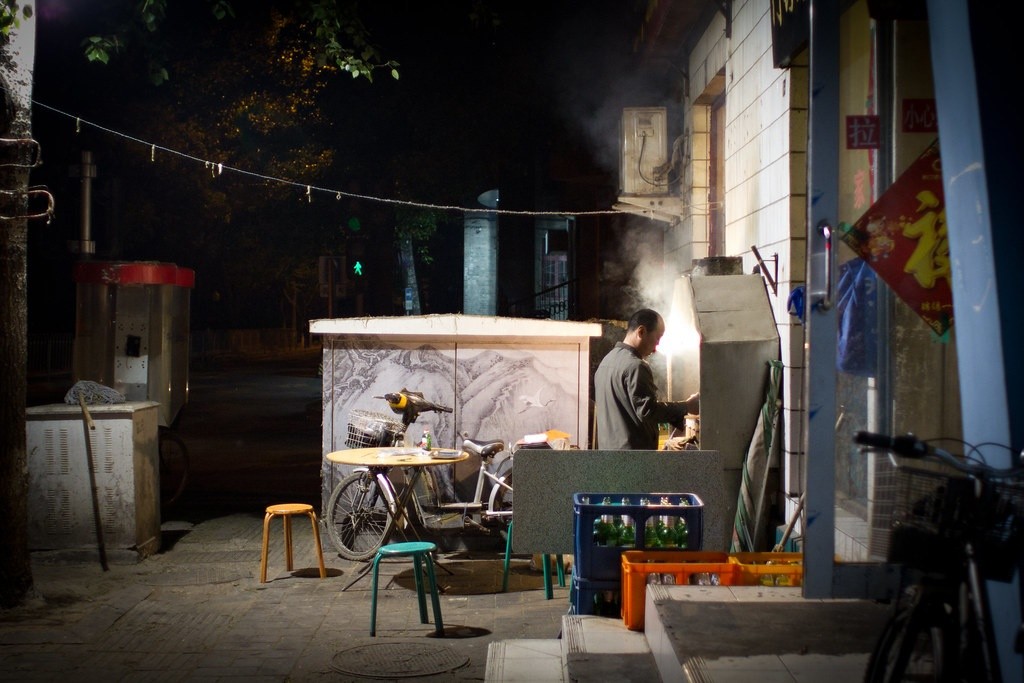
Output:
[324,388,555,560]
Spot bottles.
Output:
[581,496,691,547]
[754,560,798,586]
[421,429,431,451]
[647,559,722,584]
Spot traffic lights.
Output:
[347,212,367,240]
[347,259,369,286]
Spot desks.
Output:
[325,447,470,593]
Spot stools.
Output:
[370,542,446,638]
[501,520,565,600]
[260,503,327,582]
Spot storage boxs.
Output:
[572,493,705,580]
[621,551,737,631]
[730,552,803,587]
[570,564,621,620]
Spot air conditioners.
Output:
[618,106,669,195]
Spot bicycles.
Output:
[851,427,1023,683]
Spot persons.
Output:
[594,310,700,451]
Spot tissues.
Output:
[517,434,549,450]
